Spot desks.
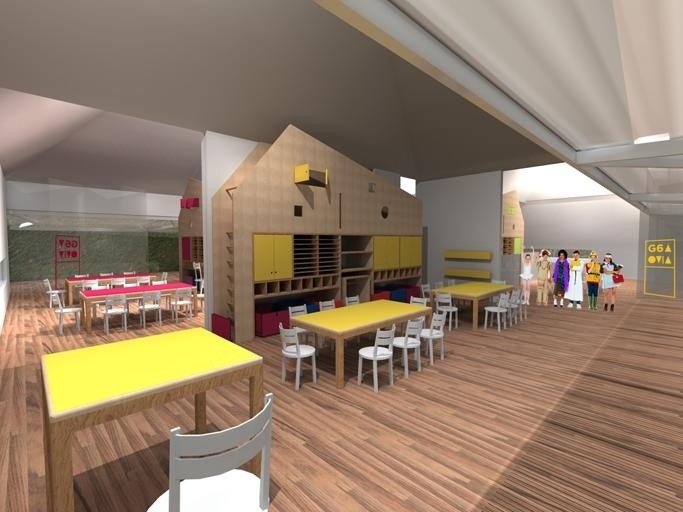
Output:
[38,326,265,512]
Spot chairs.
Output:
[43,260,205,337]
[146,392,274,512]
[279,277,528,394]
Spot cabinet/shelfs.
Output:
[252,234,294,281]
[399,236,424,269]
[373,236,399,271]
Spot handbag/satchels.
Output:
[613,274,624,283]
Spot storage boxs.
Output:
[255,303,290,338]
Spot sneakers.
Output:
[576,304,582,309]
[567,303,573,308]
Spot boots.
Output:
[559,300,564,307]
[553,299,558,307]
